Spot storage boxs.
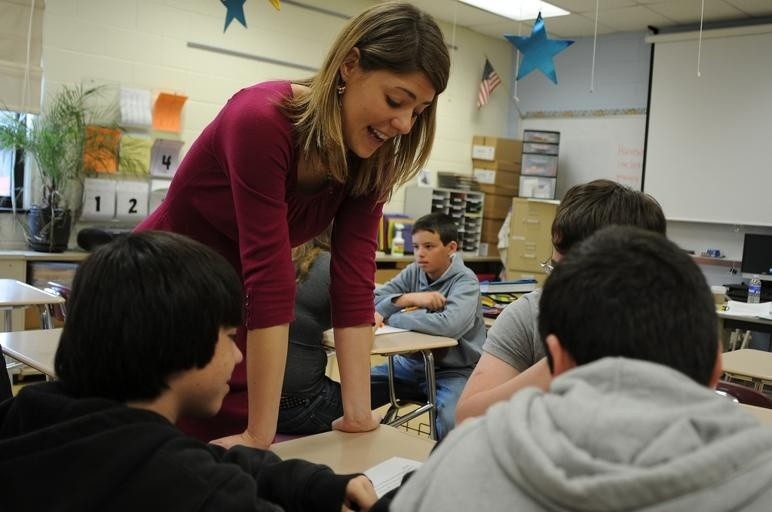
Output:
[472,129,561,286]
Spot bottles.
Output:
[390,232,404,255]
[747,273,761,311]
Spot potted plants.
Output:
[0,77,150,252]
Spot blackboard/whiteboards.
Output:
[516,113,647,206]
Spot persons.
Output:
[456,177,669,429]
[381,224,771,512]
[130,0,448,455]
[276,219,345,436]
[1,231,376,512]
[368,210,488,443]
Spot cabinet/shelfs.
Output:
[405,187,486,256]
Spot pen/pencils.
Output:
[400,306,422,313]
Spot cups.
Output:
[711,284,728,311]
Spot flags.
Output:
[474,58,504,113]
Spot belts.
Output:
[278,395,304,411]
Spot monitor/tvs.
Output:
[741,233,772,283]
